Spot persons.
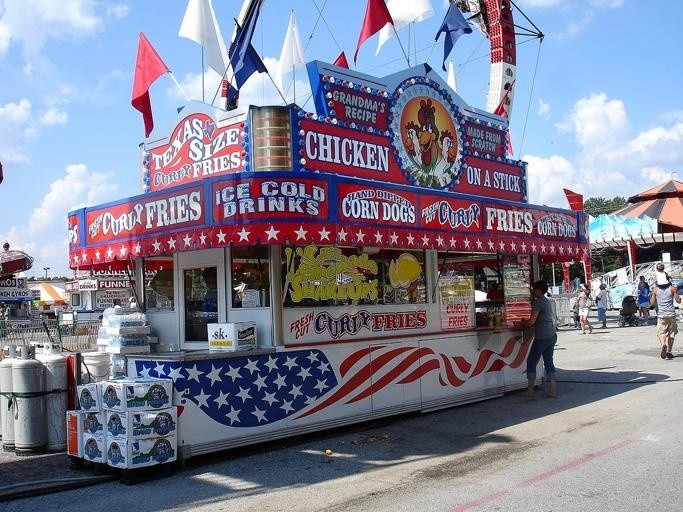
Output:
[649,272,681,360]
[637,275,649,296]
[576,283,594,335]
[200,267,216,339]
[518,279,557,398]
[595,283,610,330]
[655,263,671,289]
[637,282,650,325]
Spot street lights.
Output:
[44,267,50,279]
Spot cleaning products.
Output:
[488,305,505,327]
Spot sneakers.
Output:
[659,344,675,359]
[579,325,593,335]
[518,389,537,398]
[638,315,653,326]
[541,392,558,399]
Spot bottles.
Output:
[97,308,159,355]
[261,288,267,307]
[487,312,502,327]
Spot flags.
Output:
[226,0,261,89]
[353,0,393,66]
[447,60,461,92]
[224,19,266,106]
[374,1,436,54]
[332,50,350,70]
[176,1,239,92]
[274,14,306,80]
[494,80,517,116]
[130,31,172,140]
[229,1,252,45]
[434,2,473,74]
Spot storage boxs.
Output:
[206,321,258,353]
[65,375,177,469]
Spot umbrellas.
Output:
[28,282,71,310]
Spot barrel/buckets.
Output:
[81,352,111,382]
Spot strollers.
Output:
[615,296,640,327]
